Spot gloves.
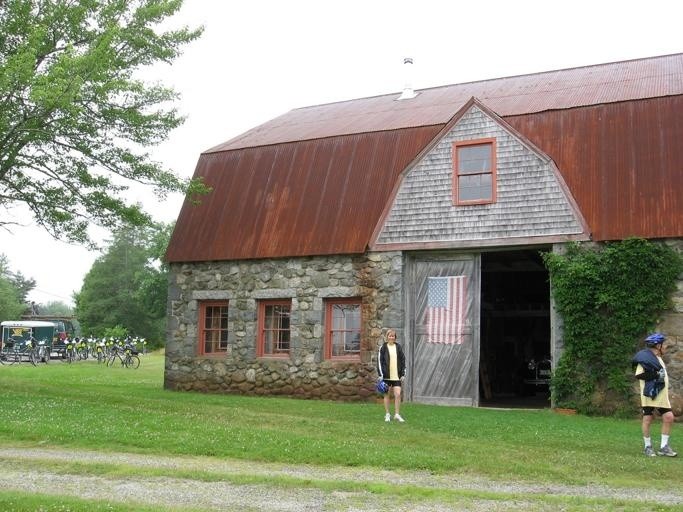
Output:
[657,368,665,378]
[400,376,405,383]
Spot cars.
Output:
[524,350,551,386]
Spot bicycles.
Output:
[0,335,142,370]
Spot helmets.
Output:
[644,334,665,343]
[377,381,389,393]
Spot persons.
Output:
[377,329,406,422]
[632,334,678,457]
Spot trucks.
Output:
[0,318,77,358]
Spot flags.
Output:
[427,276,467,345]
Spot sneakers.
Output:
[393,415,404,422]
[384,413,390,421]
[645,445,657,457]
[657,444,677,457]
